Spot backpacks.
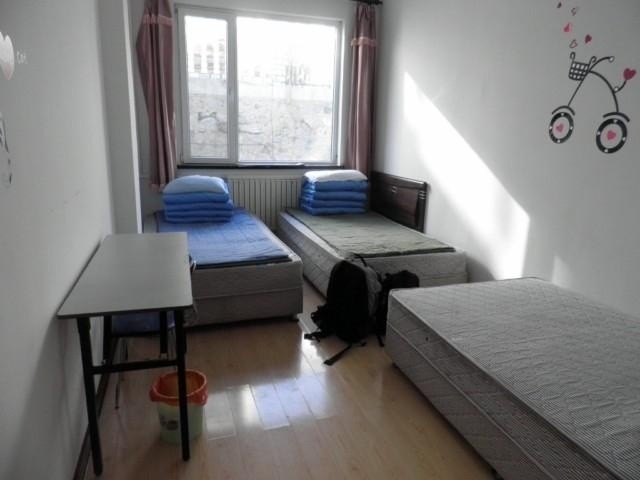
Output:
[311,253,383,344]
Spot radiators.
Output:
[226,176,304,230]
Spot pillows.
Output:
[162,175,225,193]
[304,169,368,183]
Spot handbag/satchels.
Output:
[376,270,419,346]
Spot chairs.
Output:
[104,252,197,409]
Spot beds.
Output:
[273,207,466,314]
[384,276,640,480]
[142,205,303,332]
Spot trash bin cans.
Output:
[150,369,207,446]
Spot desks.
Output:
[55,232,193,476]
